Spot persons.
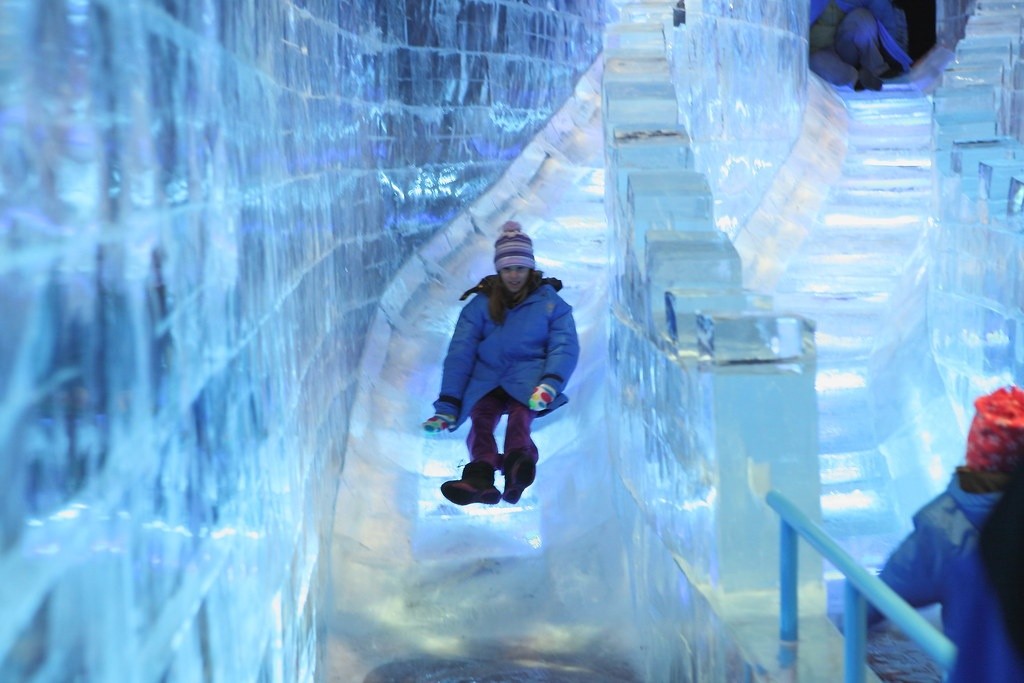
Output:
[425,222,580,506]
[807,1,910,93]
[868,387,1024,683]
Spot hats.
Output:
[494,221,534,272]
[967,387,1024,470]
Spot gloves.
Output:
[423,415,451,431]
[528,384,555,411]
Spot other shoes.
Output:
[502,457,536,504]
[441,461,502,505]
